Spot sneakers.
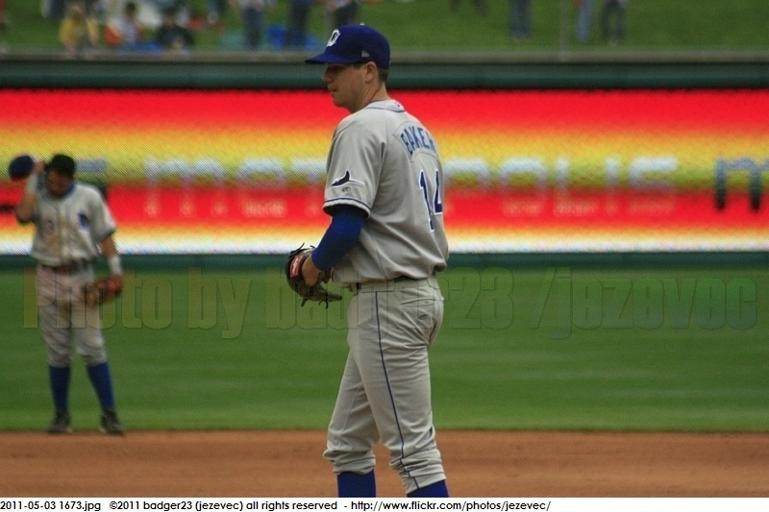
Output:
[99,408,123,434]
[47,413,72,434]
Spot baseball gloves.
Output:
[287,243,345,307]
[81,274,123,307]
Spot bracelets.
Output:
[108,252,122,278]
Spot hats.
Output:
[305,23,390,69]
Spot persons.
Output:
[285,23,457,497]
[1,0,630,62]
[9,153,124,433]
